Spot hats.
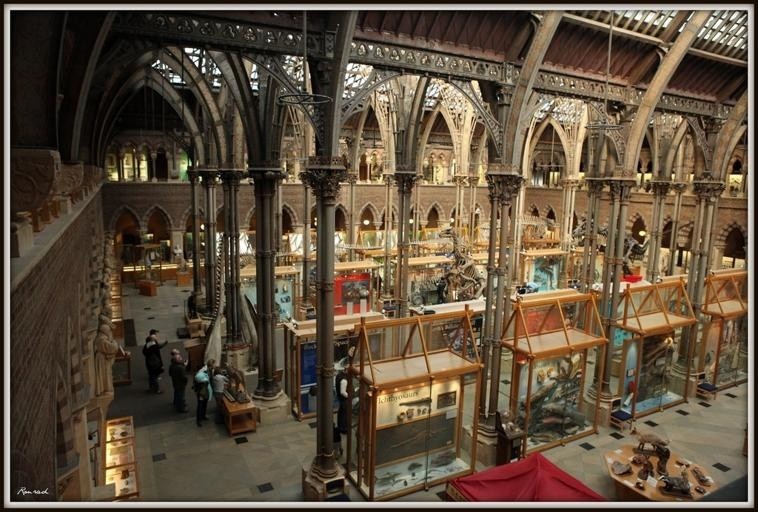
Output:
[150,329,160,334]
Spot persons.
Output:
[191,358,229,427]
[142,329,189,413]
[188,292,197,319]
[438,277,448,303]
[337,364,352,436]
[332,423,344,459]
[121,246,129,264]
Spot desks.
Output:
[605,443,717,502]
[216,383,257,436]
